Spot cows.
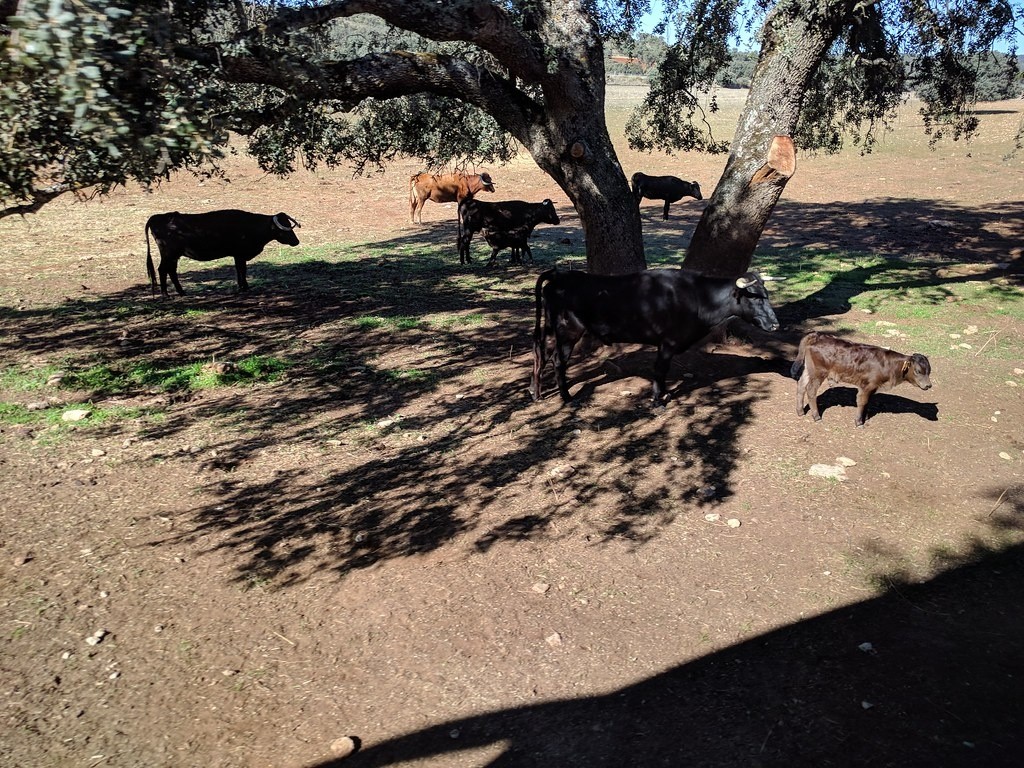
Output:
[145,209,301,299]
[528,268,781,412]
[631,172,703,220]
[790,331,933,429]
[409,171,560,268]
